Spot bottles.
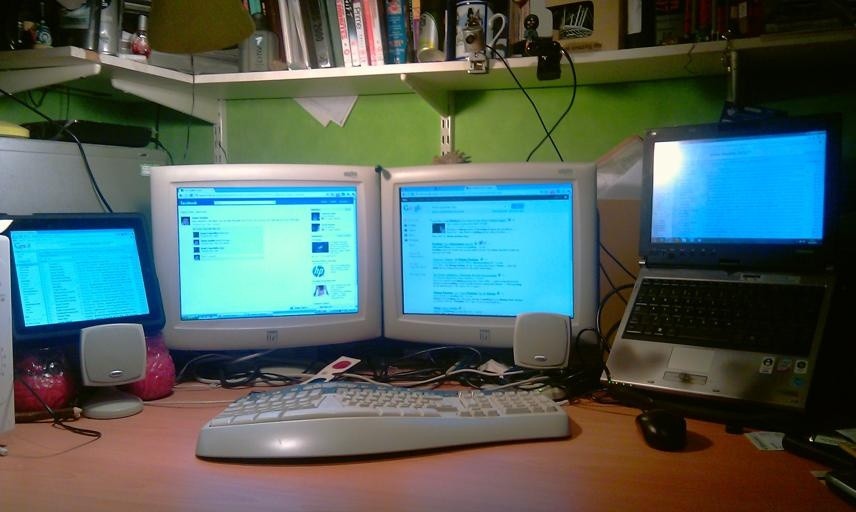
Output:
[129,12,153,56]
[30,2,54,44]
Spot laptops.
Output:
[599,118,837,417]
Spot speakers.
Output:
[513,311,568,401]
[80,325,155,421]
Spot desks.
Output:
[3,370,856,512]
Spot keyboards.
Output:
[196,383,573,463]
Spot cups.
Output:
[454,0,508,60]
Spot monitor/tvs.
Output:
[150,168,379,349]
[379,167,599,351]
[1,210,166,342]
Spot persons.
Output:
[522,13,540,58]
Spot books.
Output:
[626,0,856,49]
[276,0,425,70]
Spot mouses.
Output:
[633,407,689,449]
